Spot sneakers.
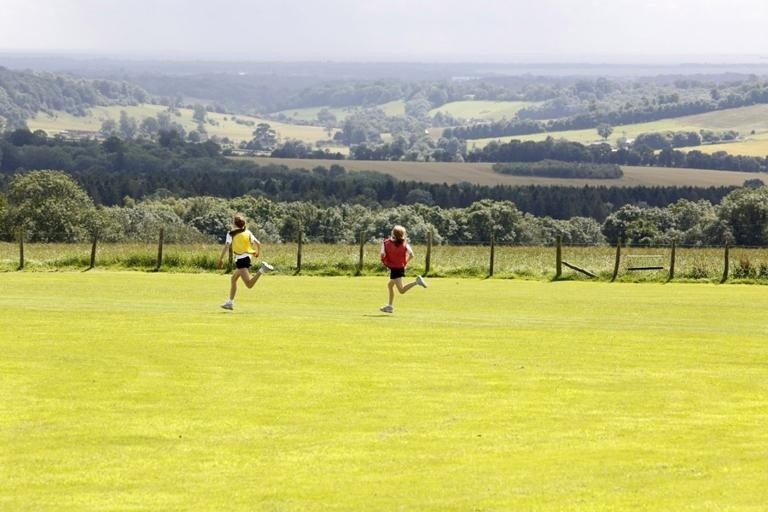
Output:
[261,261,274,271]
[220,302,234,309]
[379,305,394,313]
[415,275,428,289]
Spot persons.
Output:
[378,224,428,313]
[215,215,274,311]
